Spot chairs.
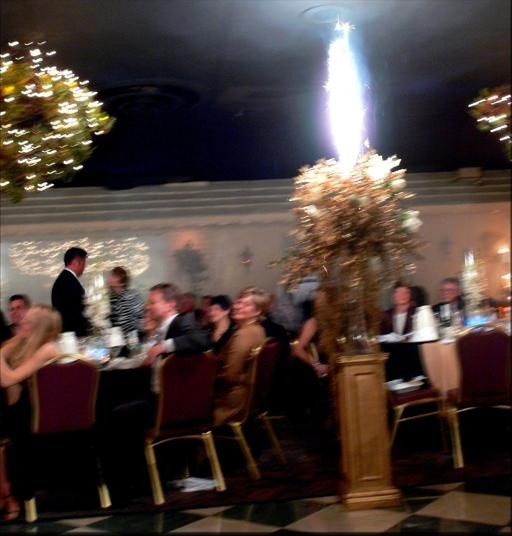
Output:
[288,316,511,474]
[1,332,281,524]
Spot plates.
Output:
[390,381,425,394]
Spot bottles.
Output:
[63,331,78,355]
[109,327,129,357]
[416,305,439,342]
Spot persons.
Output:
[0,293,74,521]
[94,284,283,495]
[381,277,466,335]
[106,267,145,338]
[290,273,331,407]
[51,247,91,338]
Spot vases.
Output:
[336,250,375,359]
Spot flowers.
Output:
[271,146,423,299]
